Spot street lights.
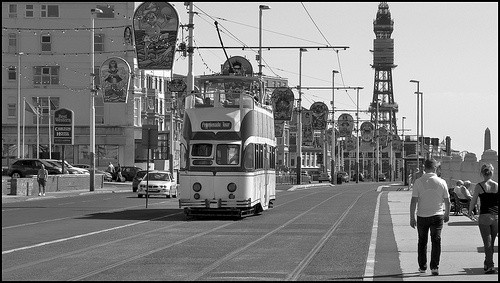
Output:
[16,52,24,159]
[296,48,308,185]
[331,70,340,185]
[258,4,271,77]
[89,7,104,192]
[410,79,420,169]
[414,91,424,144]
[401,116,406,182]
[356,86,363,183]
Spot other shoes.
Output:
[484,264,488,271]
[484,268,496,274]
[43,193,45,196]
[418,268,425,273]
[431,269,438,275]
[39,192,41,196]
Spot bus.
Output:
[178,93,279,218]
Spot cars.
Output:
[133,169,151,192]
[111,165,147,181]
[138,170,178,198]
[2,158,113,182]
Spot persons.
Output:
[117,164,121,175]
[468,163,498,273]
[37,164,48,196]
[454,180,478,215]
[191,91,203,104]
[108,162,113,173]
[229,152,238,164]
[410,159,450,275]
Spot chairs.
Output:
[448,188,477,216]
